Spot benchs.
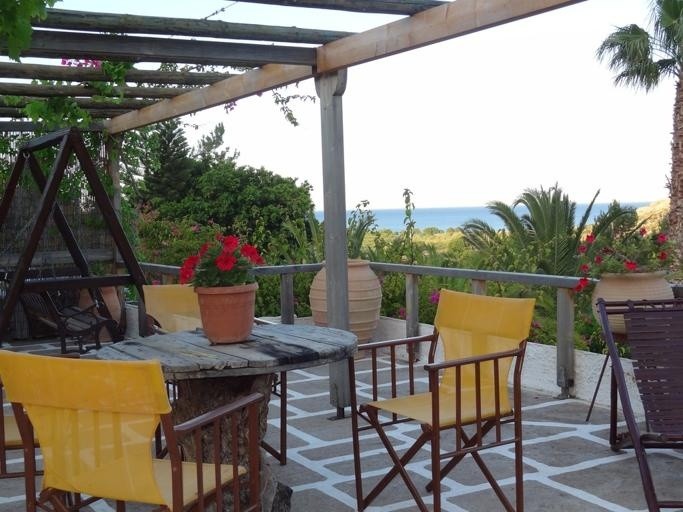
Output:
[18,291,106,350]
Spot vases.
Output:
[192,280,258,345]
[592,268,674,333]
[309,260,382,360]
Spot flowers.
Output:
[570,224,672,295]
[178,234,262,287]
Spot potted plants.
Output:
[78,262,121,343]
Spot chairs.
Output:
[593,297,683,512]
[347,288,536,512]
[141,281,204,330]
[0,384,45,478]
[0,348,266,512]
